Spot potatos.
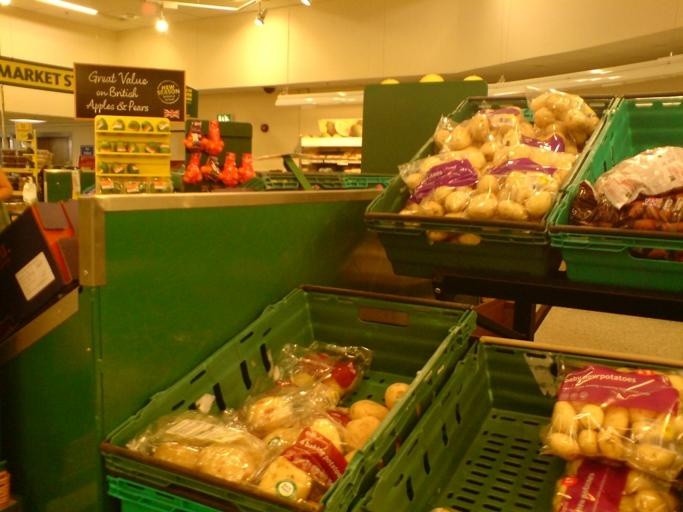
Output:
[154,371,422,501]
[537,367,683,512]
[396,91,599,247]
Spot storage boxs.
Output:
[0,199,80,341]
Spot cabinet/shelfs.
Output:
[94,115,173,194]
[3,167,41,196]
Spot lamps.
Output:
[33,1,100,16]
[155,1,168,33]
[274,0,364,108]
[253,3,271,26]
